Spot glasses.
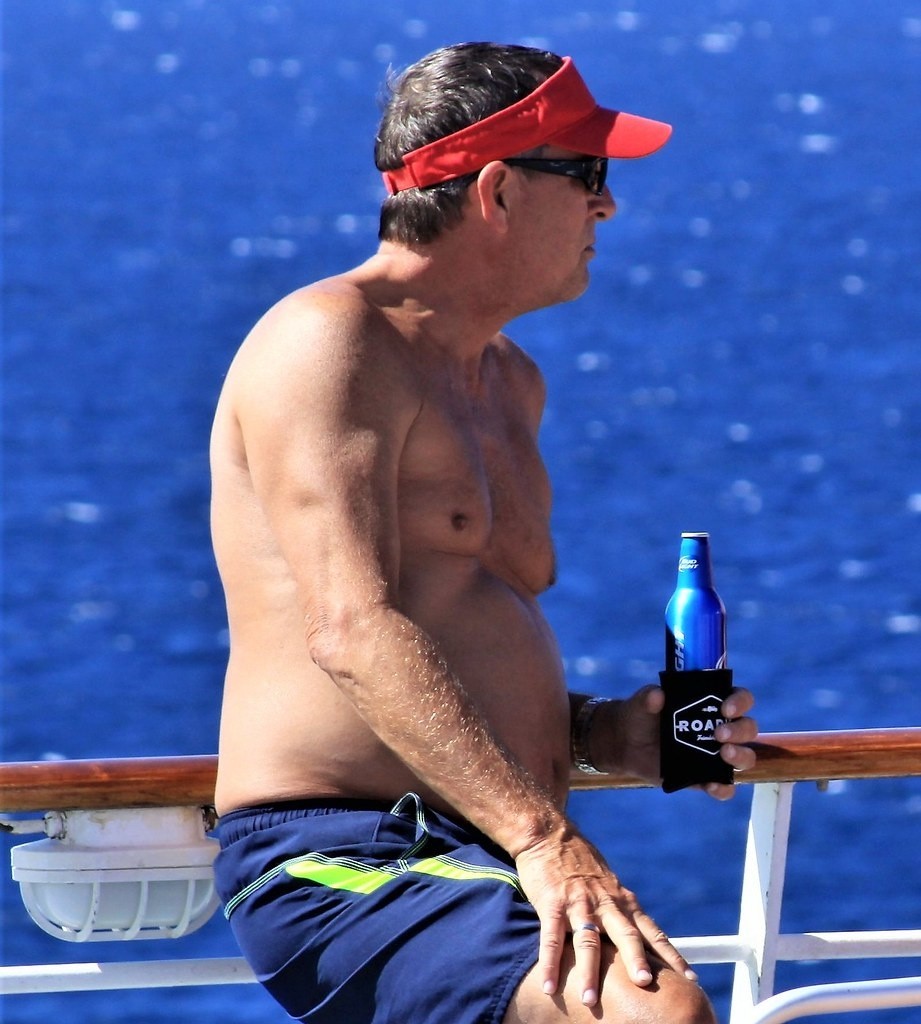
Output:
[456,150,611,198]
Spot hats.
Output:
[381,47,675,202]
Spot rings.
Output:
[571,923,600,934]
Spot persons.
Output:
[202,41,766,1024]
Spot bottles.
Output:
[663,531,728,671]
